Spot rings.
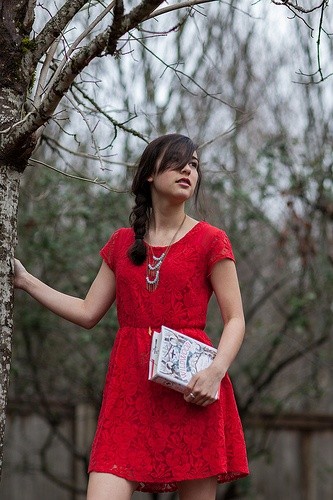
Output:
[190,393,196,401]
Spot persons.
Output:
[12,134,251,500]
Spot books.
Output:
[148,326,221,407]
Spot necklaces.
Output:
[145,214,187,292]
